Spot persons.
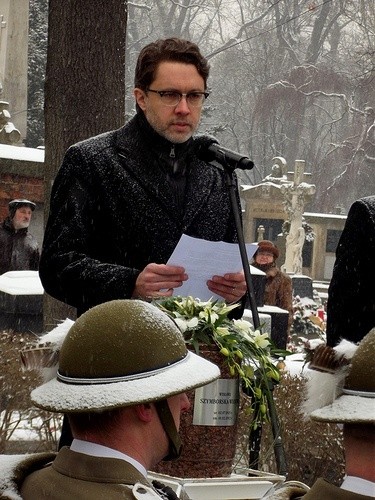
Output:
[253,240,293,313]
[263,328,375,500]
[0,198,43,331]
[39,38,248,448]
[325,196,375,348]
[0,299,221,500]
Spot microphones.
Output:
[192,134,254,170]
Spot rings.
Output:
[231,288,234,294]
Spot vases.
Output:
[152,344,241,477]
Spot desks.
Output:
[146,465,286,500]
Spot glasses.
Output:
[143,87,210,108]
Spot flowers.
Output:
[153,292,287,431]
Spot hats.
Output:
[253,240,279,262]
[8,199,36,211]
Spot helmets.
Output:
[30,300,222,413]
[307,325,375,426]
